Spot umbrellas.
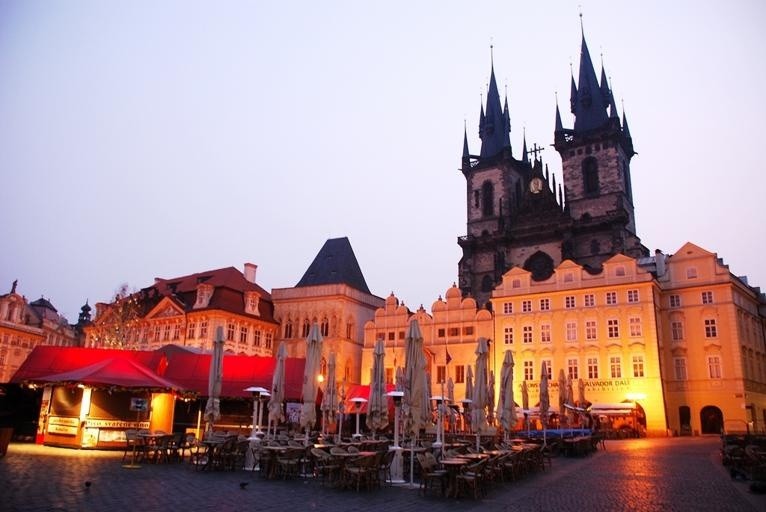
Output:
[299,322,320,444]
[496,347,519,442]
[366,339,390,433]
[393,360,586,428]
[267,339,288,440]
[319,354,337,437]
[470,337,490,453]
[201,326,225,439]
[402,318,426,485]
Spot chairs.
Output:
[129,419,606,498]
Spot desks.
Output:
[44,415,150,449]
